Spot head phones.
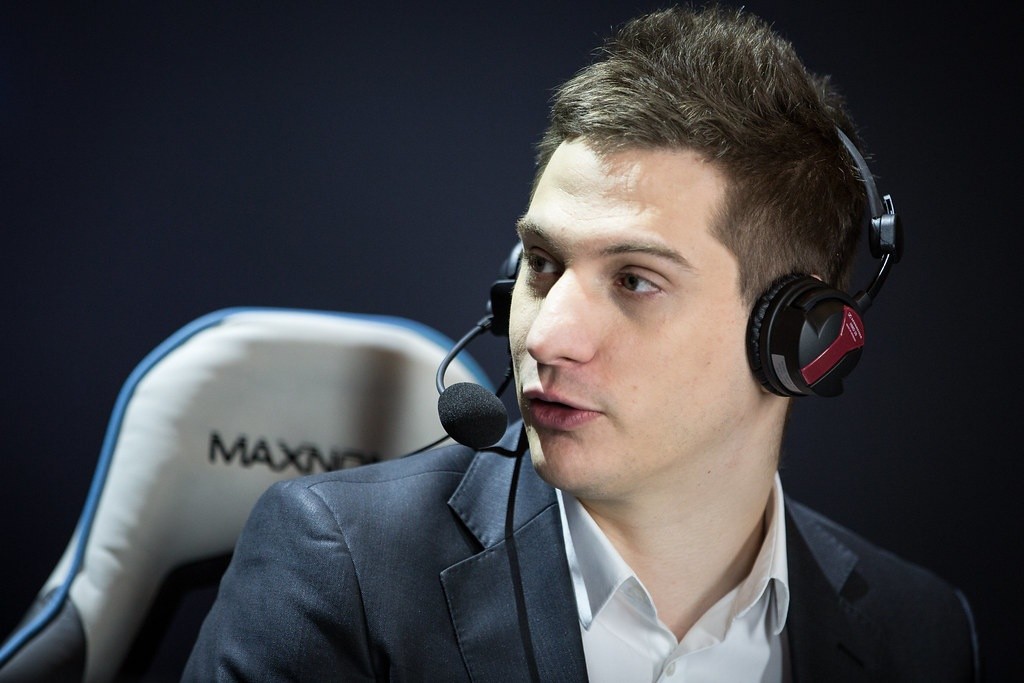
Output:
[488,121,897,398]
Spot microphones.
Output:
[436,301,508,448]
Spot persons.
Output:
[182,9,975,683]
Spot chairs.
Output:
[0,304,511,683]
